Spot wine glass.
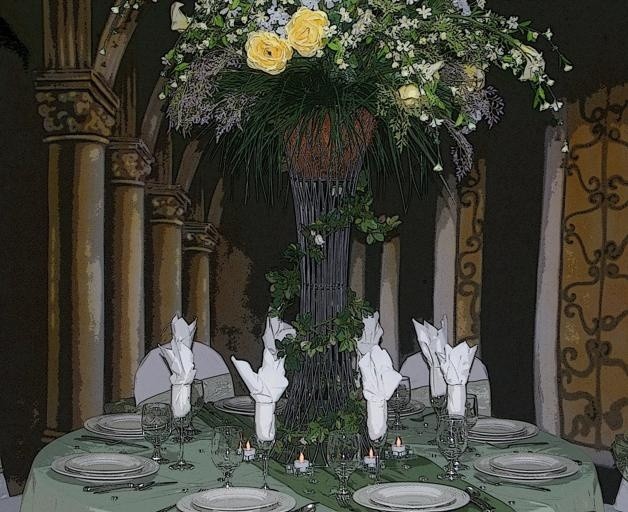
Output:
[141,401,173,464]
[326,429,362,498]
[186,378,205,436]
[437,414,467,481]
[210,425,242,488]
[464,393,479,453]
[389,375,412,431]
[366,399,389,486]
[168,383,196,471]
[426,366,448,446]
[253,401,280,492]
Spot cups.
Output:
[447,382,467,415]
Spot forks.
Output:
[336,494,362,512]
[486,441,549,449]
[473,472,551,492]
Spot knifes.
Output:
[81,434,149,448]
[93,481,178,493]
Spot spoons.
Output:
[465,486,496,512]
[82,480,156,491]
[301,503,316,512]
[73,437,122,445]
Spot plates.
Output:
[214,395,288,416]
[51,452,161,483]
[176,485,297,512]
[467,416,540,444]
[387,397,426,418]
[352,480,471,512]
[472,452,579,480]
[83,412,146,440]
